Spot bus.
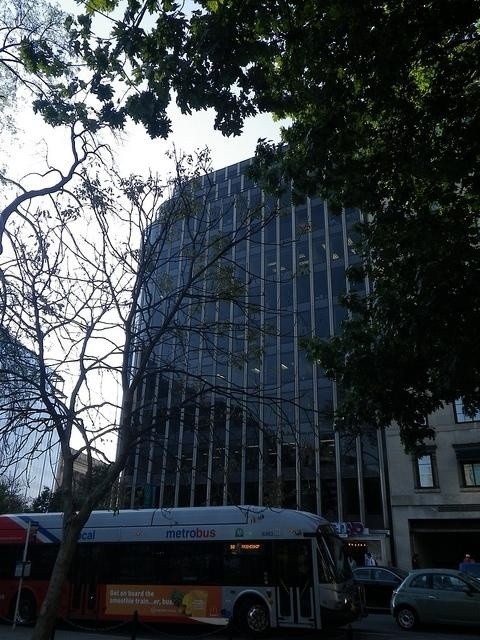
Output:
[0,505,364,639]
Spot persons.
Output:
[464,553,475,563]
[348,556,356,570]
[365,552,377,566]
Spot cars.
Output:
[352,565,409,609]
[390,568,480,632]
